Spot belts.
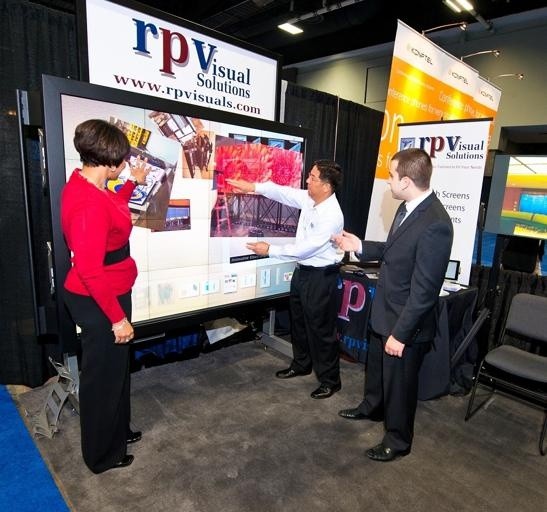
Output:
[295,262,339,273]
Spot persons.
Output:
[61,119,153,474]
[332,148,454,461]
[225,159,345,399]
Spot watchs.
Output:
[128,176,138,186]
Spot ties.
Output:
[391,204,408,236]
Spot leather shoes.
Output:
[310,382,342,400]
[275,362,313,379]
[338,404,386,423]
[365,441,411,462]
[109,430,143,445]
[91,454,134,474]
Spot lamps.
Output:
[491,70,527,83]
[420,20,467,35]
[460,47,501,64]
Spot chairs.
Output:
[463,292,546,456]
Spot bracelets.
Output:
[112,317,127,332]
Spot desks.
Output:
[336,260,479,401]
